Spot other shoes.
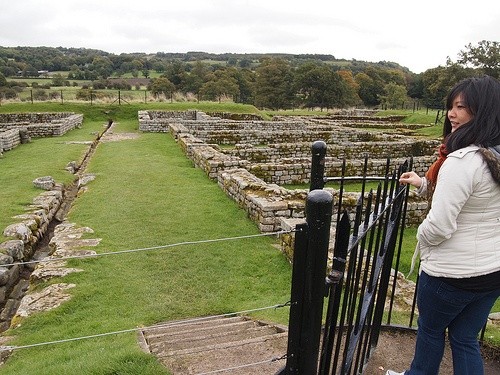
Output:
[385,369,405,375]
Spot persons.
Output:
[384,73,500,375]
